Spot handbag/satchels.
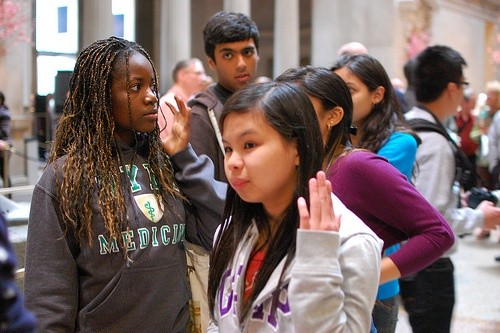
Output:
[182,238,213,333]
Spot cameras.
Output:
[468,187,498,209]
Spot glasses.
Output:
[452,80,470,89]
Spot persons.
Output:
[186,12,260,182]
[339,42,369,56]
[332,55,422,333]
[454,80,500,239]
[206,81,385,332]
[399,45,500,333]
[24,36,228,333]
[157,58,213,141]
[277,66,455,333]
